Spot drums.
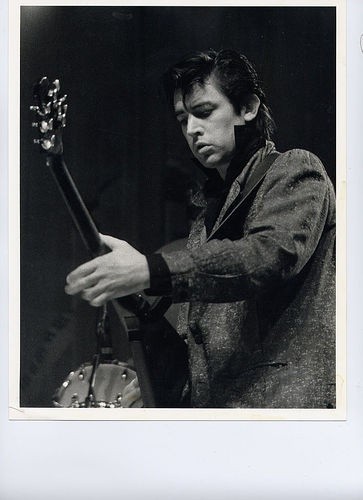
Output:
[52,359,144,408]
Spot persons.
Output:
[62,42,336,410]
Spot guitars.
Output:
[30,75,190,408]
